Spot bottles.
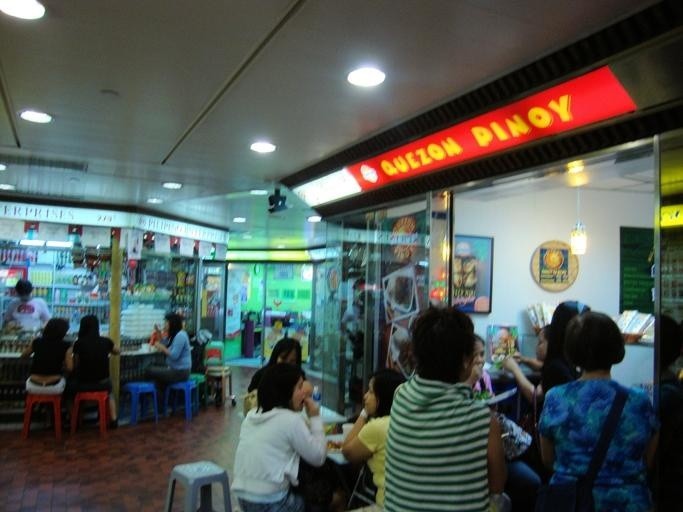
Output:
[312,386,321,407]
[32,287,52,304]
[0,388,26,410]
[54,307,109,326]
[60,289,67,305]
[121,340,141,352]
[55,289,60,305]
[120,356,146,382]
[1,341,28,353]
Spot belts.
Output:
[29,379,60,386]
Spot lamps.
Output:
[565,161,588,257]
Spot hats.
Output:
[549,300,591,338]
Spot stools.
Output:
[162,380,199,425]
[22,393,63,442]
[204,366,233,408]
[70,392,109,438]
[186,371,208,413]
[119,382,159,429]
[164,459,231,512]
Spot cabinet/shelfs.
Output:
[3,244,198,337]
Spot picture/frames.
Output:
[447,234,495,314]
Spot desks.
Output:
[1,331,160,433]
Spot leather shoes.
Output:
[109,418,119,430]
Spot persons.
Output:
[540,300,591,397]
[2,279,51,336]
[230,361,328,512]
[501,324,550,401]
[20,317,74,414]
[381,307,511,512]
[537,312,661,511]
[145,312,193,385]
[248,337,307,409]
[340,366,409,512]
[466,335,542,494]
[66,315,122,430]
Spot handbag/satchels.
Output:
[534,387,629,512]
